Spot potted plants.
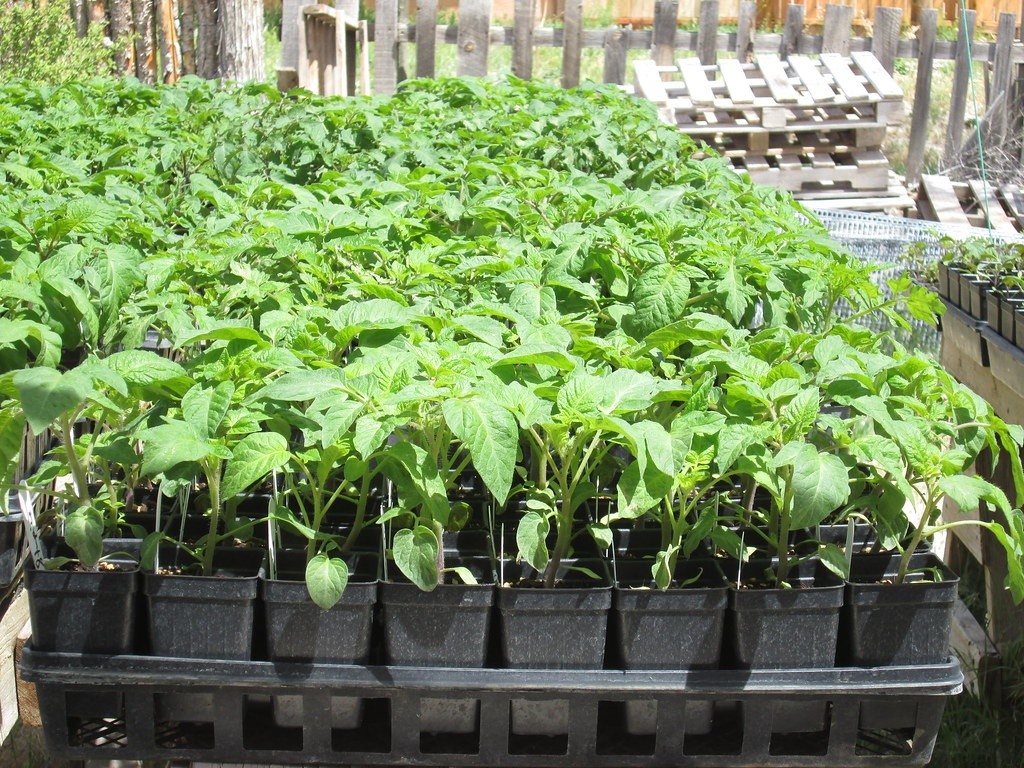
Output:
[0,74,1024,735]
